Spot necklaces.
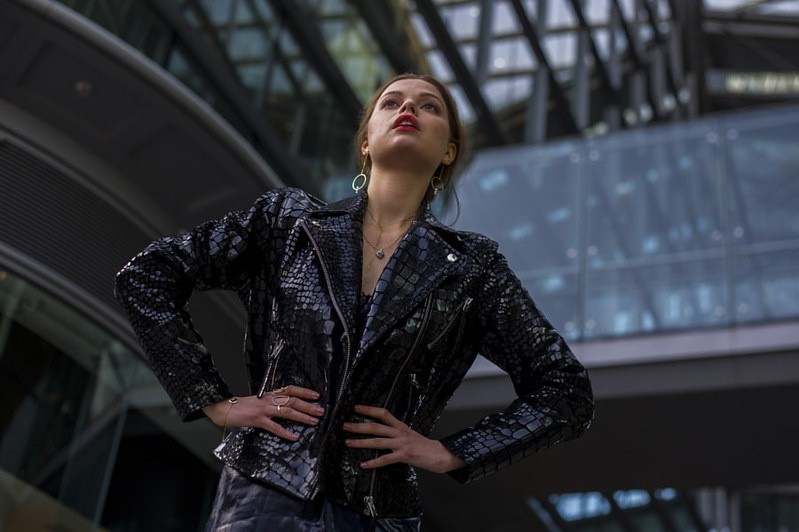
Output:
[361,204,416,259]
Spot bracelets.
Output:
[222,397,239,442]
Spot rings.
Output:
[272,395,290,406]
[273,386,287,393]
[277,405,282,418]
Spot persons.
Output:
[112,73,595,532]
